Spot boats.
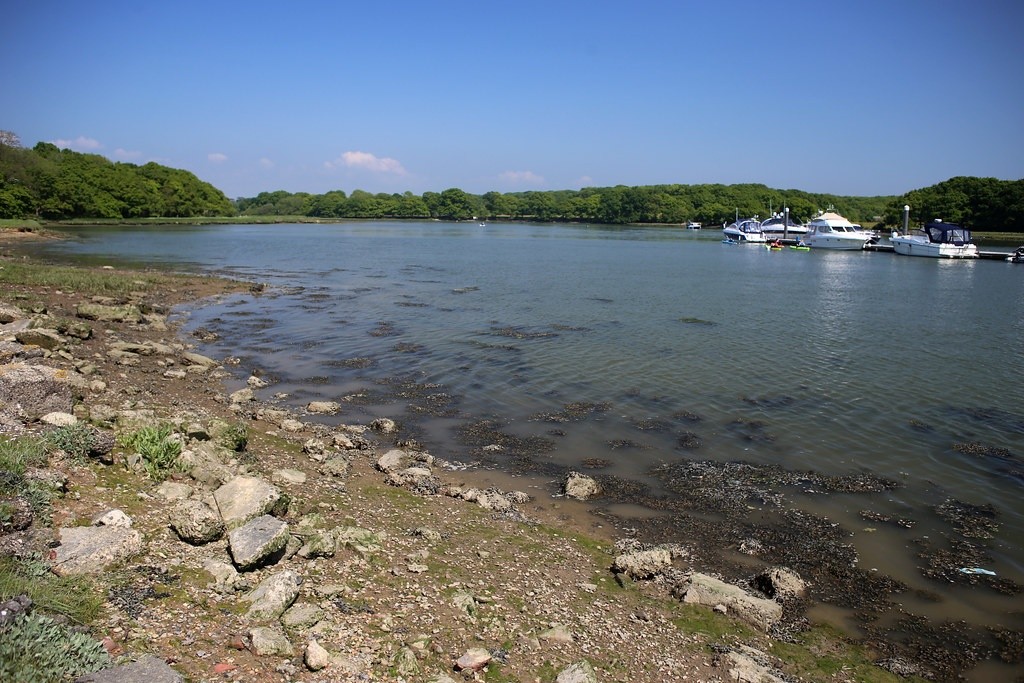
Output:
[889,205,981,258]
[722,206,882,250]
[687,219,702,229]
[1004,246,1024,264]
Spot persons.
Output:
[771,239,805,247]
